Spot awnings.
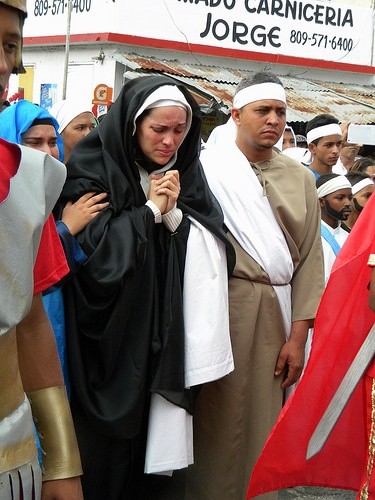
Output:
[111,50,375,127]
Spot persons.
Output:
[0,100,11,113]
[272,123,298,153]
[316,173,352,291]
[0,100,110,471]
[294,135,308,149]
[352,237,375,500]
[170,72,326,499]
[50,74,238,500]
[348,157,375,184]
[339,171,375,237]
[47,98,99,165]
[0,0,87,500]
[301,114,344,180]
[332,122,366,176]
